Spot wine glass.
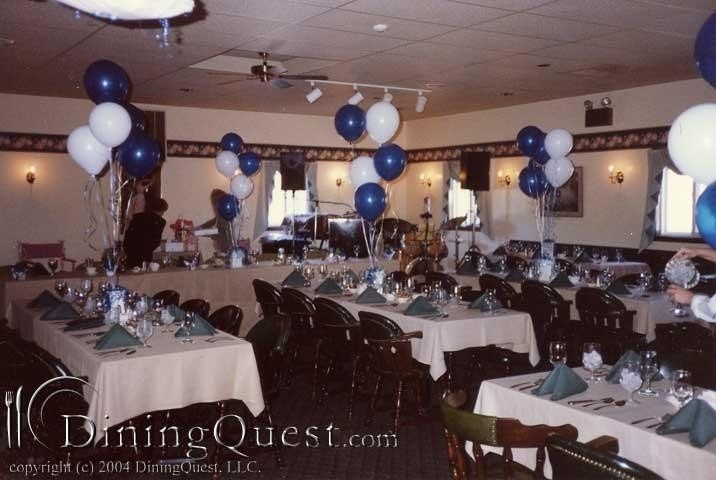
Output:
[547,340,567,369]
[671,278,688,316]
[135,319,153,347]
[617,360,642,407]
[180,312,196,342]
[671,368,692,410]
[639,350,658,397]
[476,256,667,300]
[159,304,174,332]
[582,343,602,384]
[303,263,497,316]
[503,243,622,263]
[54,279,146,325]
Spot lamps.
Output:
[305,80,428,113]
[496,171,511,188]
[420,173,433,188]
[608,165,624,186]
[335,175,342,186]
[26,165,34,184]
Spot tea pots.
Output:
[665,256,700,289]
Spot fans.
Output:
[217,54,328,89]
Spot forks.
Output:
[202,335,234,343]
[518,378,542,391]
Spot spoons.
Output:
[568,397,611,405]
[593,401,624,411]
[582,399,615,407]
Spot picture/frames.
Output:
[540,166,584,216]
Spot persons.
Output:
[666,245,715,324]
[123,197,171,271]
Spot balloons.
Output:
[373,144,407,181]
[512,123,575,201]
[692,181,716,252]
[65,56,163,184]
[335,104,368,143]
[354,183,386,222]
[692,11,716,89]
[363,102,400,145]
[211,130,260,222]
[349,156,380,190]
[665,101,716,186]
